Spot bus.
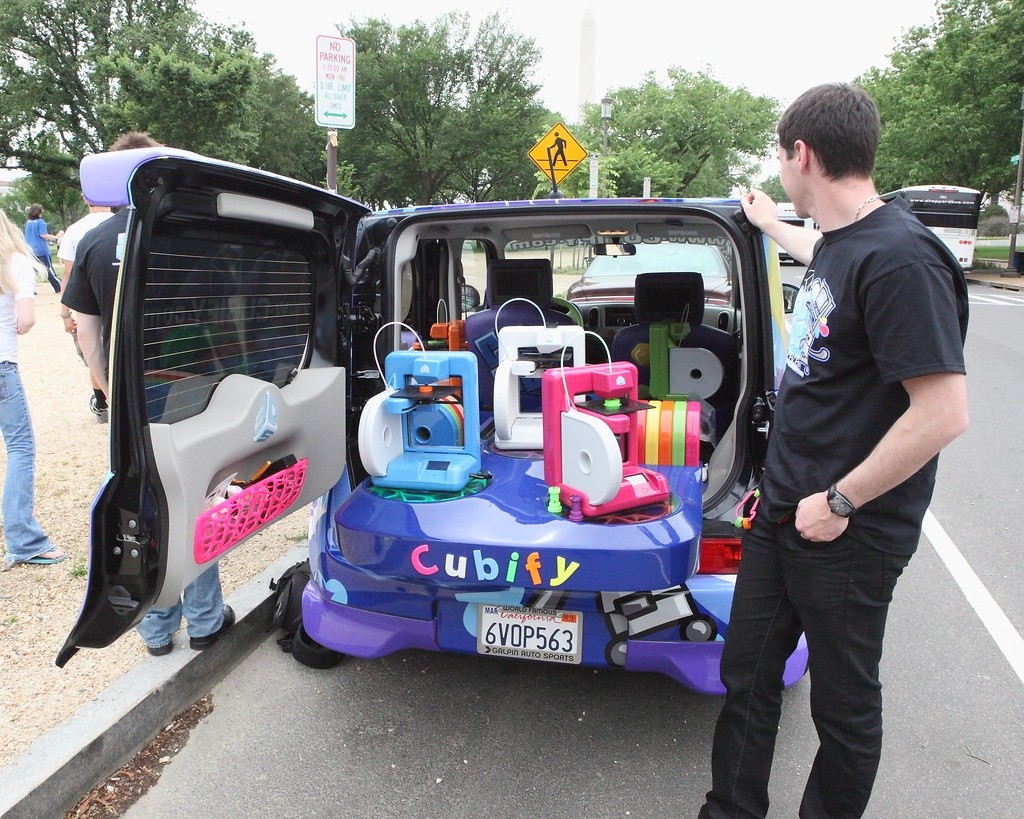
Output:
[880,184,983,276]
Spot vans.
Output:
[771,203,821,268]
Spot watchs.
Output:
[827,484,857,518]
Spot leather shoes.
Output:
[147,639,173,656]
[190,603,235,650]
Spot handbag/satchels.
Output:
[269,557,343,669]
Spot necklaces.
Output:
[846,194,881,222]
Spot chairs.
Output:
[610,271,735,387]
[466,259,575,410]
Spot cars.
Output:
[50,145,808,705]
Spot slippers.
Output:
[5,546,67,564]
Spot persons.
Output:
[0,131,234,655]
[695,83,972,819]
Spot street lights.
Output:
[598,93,615,198]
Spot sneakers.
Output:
[90,394,108,424]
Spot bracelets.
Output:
[60,312,72,319]
